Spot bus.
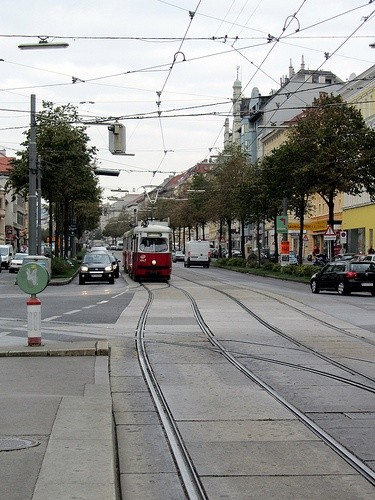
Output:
[122,217,172,283]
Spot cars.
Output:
[110,254,121,279]
[172,251,185,263]
[9,253,28,273]
[209,248,242,259]
[78,252,116,284]
[334,253,375,262]
[248,252,298,266]
[309,260,375,295]
[90,240,123,253]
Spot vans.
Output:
[0,245,14,269]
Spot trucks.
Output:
[184,240,209,268]
